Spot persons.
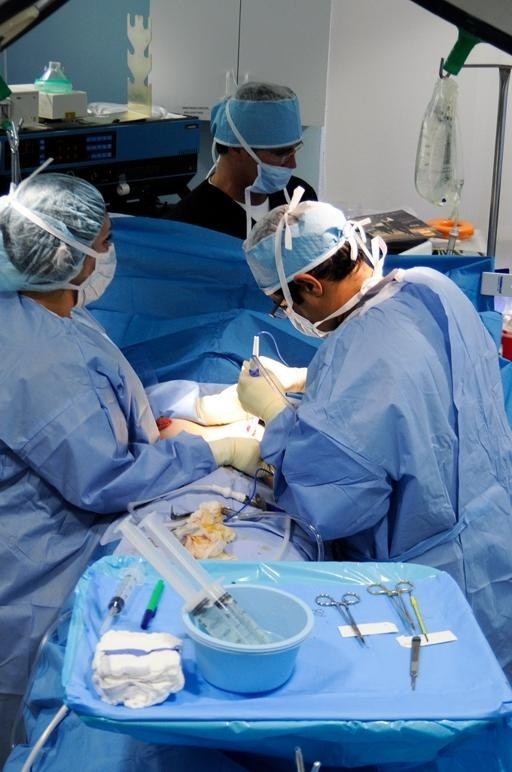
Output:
[161,80,304,240]
[0,173,277,772]
[155,416,265,442]
[237,199,512,680]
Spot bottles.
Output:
[34,59,74,93]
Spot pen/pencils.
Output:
[141,580,164,629]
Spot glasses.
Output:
[270,297,289,319]
[276,142,303,161]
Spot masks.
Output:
[282,282,338,337]
[77,244,117,304]
[236,147,294,194]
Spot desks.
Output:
[14,555,512,772]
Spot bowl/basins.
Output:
[182,583,317,694]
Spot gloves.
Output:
[209,438,275,477]
[238,361,290,423]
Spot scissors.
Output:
[367,580,417,637]
[315,592,367,648]
